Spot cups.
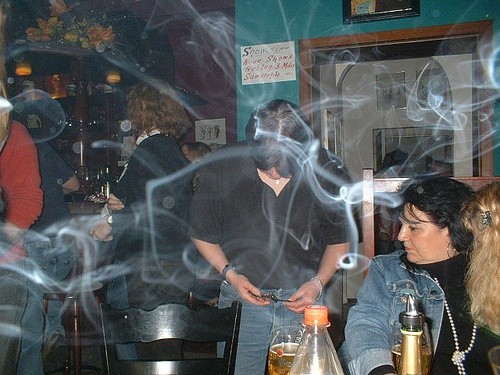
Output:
[389,322,432,375]
[267,325,305,375]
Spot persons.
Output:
[337,149,500,375]
[88,77,219,360]
[0,120,80,375]
[188,99,353,375]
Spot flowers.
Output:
[25,0,116,53]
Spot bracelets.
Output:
[310,277,323,301]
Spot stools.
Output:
[43,282,105,375]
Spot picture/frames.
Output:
[376,70,406,110]
[416,69,447,108]
[341,0,420,24]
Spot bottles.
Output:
[286,304,345,375]
[399,293,425,375]
[74,166,110,199]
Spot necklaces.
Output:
[434,276,476,375]
[275,180,280,185]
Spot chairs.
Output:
[99,302,242,375]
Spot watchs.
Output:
[221,264,235,285]
[107,215,113,228]
